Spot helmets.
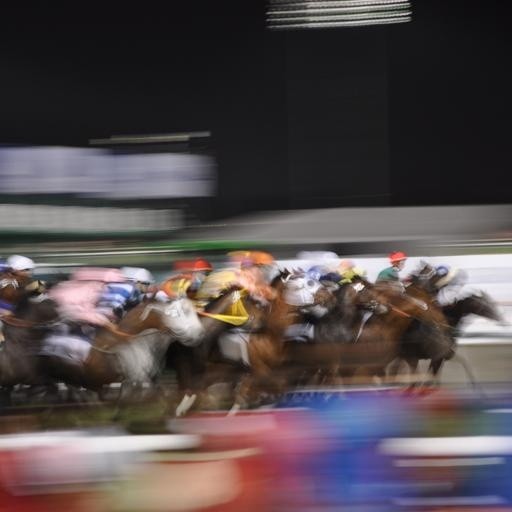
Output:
[7,255,35,271]
[173,251,274,273]
[70,265,156,285]
[390,251,407,263]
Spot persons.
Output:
[1,247,416,391]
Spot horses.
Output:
[0,265,503,424]
